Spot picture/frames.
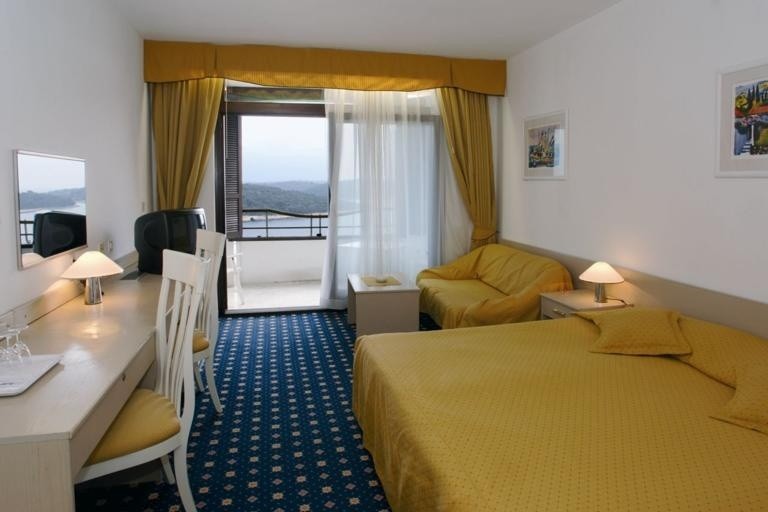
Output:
[520,106,570,180]
[714,57,768,177]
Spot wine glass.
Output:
[0,324,32,372]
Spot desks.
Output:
[347,272,420,340]
[337,241,399,295]
[0,250,205,512]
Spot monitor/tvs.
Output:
[134,207,207,275]
[32,211,86,257]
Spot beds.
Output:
[352,308,768,512]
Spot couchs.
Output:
[416,244,574,329]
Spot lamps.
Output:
[579,261,624,303]
[60,249,123,304]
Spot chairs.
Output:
[228,239,245,308]
[74,249,210,512]
[155,228,227,418]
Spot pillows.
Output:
[661,315,768,390]
[710,363,768,434]
[585,309,693,356]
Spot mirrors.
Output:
[13,149,86,270]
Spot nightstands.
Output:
[540,289,632,320]
